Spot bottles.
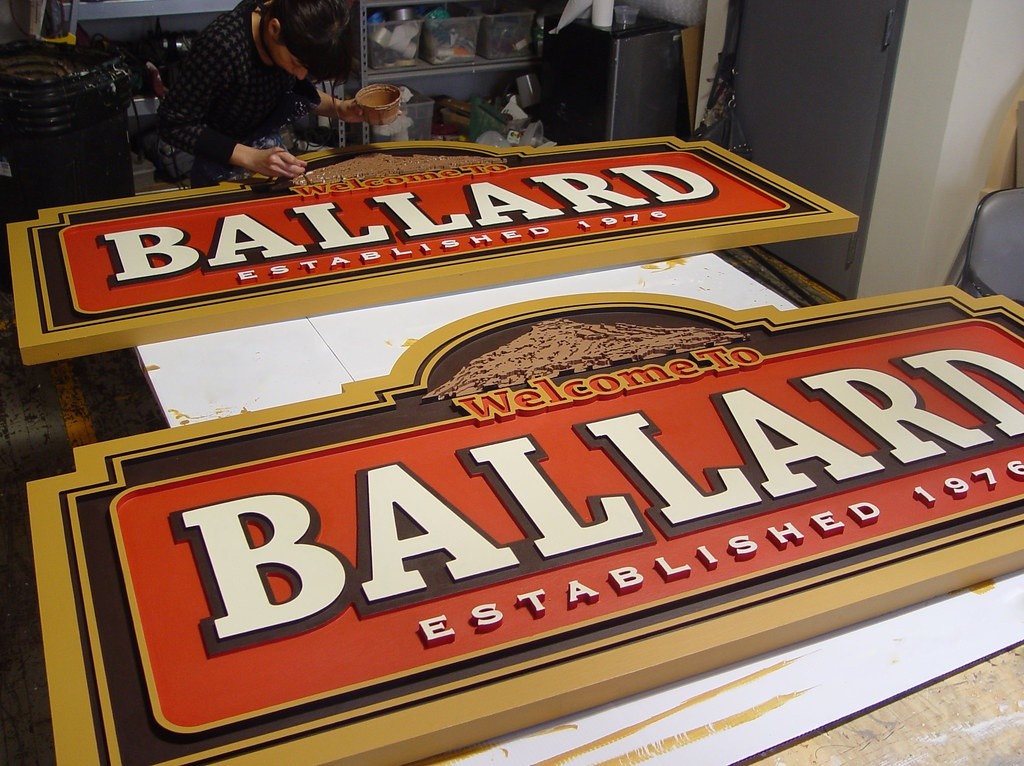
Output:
[532,18,544,55]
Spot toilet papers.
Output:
[549,0,616,35]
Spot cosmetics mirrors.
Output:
[36,0,77,47]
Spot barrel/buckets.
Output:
[0,40,135,222]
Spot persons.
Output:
[157,0,401,179]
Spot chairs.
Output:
[964,187,1024,307]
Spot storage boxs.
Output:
[367,15,425,70]
[370,84,436,143]
[419,3,484,66]
[441,106,470,130]
[476,3,536,60]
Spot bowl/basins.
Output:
[615,6,639,24]
[356,84,401,126]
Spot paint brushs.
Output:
[268,127,312,185]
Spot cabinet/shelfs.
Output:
[535,15,688,145]
[46,0,345,177]
[348,2,536,146]
